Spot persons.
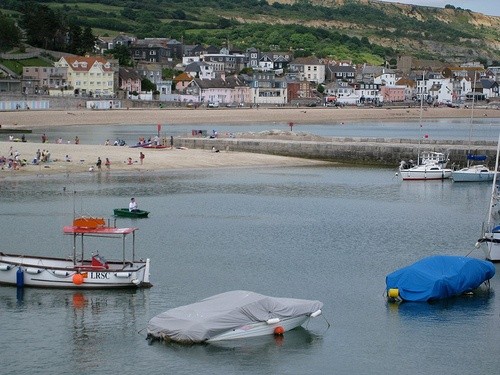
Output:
[129,198,139,212]
[0,129,221,170]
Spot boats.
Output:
[385,253,495,303]
[396,150,453,180]
[0,216,153,291]
[449,155,498,181]
[147,288,324,342]
[112,207,152,218]
[478,136,500,263]
